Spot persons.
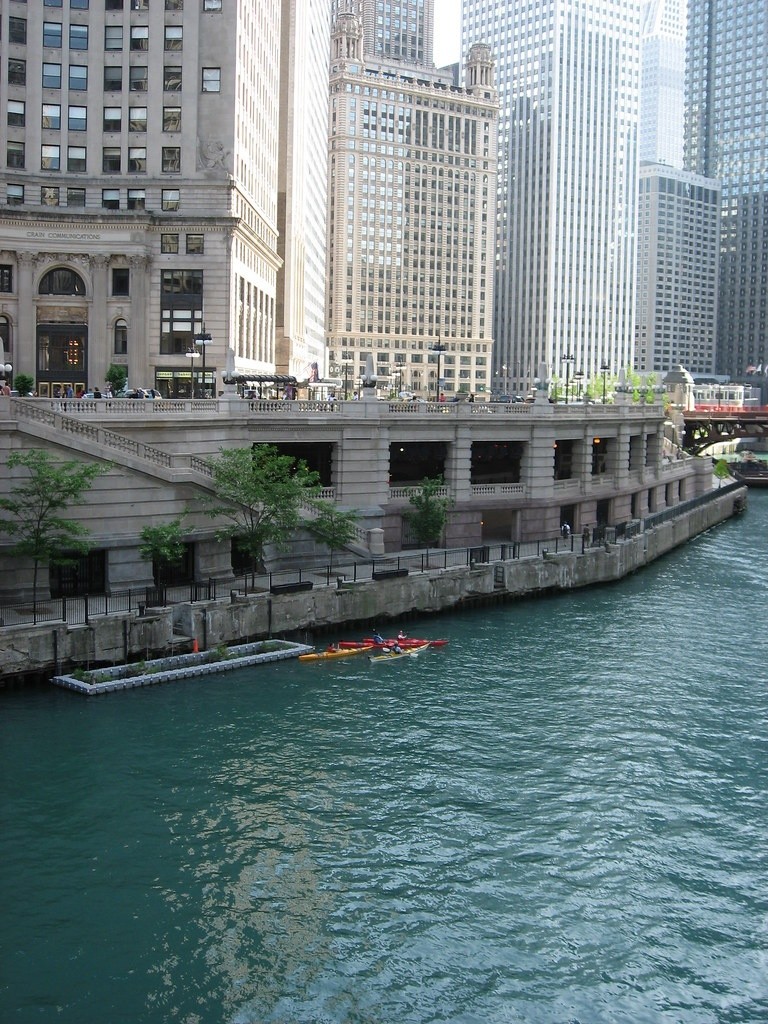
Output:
[353,392,358,400]
[584,524,590,548]
[66,385,73,398]
[327,642,336,653]
[128,388,156,399]
[373,632,384,643]
[328,393,336,401]
[392,641,404,654]
[397,630,407,640]
[2,382,12,396]
[468,394,474,402]
[410,396,418,403]
[53,385,63,398]
[439,393,446,402]
[80,387,101,399]
[561,521,570,547]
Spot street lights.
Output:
[599,366,612,404]
[431,345,447,402]
[0,364,13,378]
[574,373,584,402]
[561,354,575,403]
[185,347,201,399]
[391,371,401,397]
[194,333,213,398]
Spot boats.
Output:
[297,646,373,660]
[727,450,768,485]
[367,643,429,662]
[333,641,424,649]
[362,637,449,646]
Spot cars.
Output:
[82,394,107,398]
[116,389,162,399]
[11,391,34,397]
